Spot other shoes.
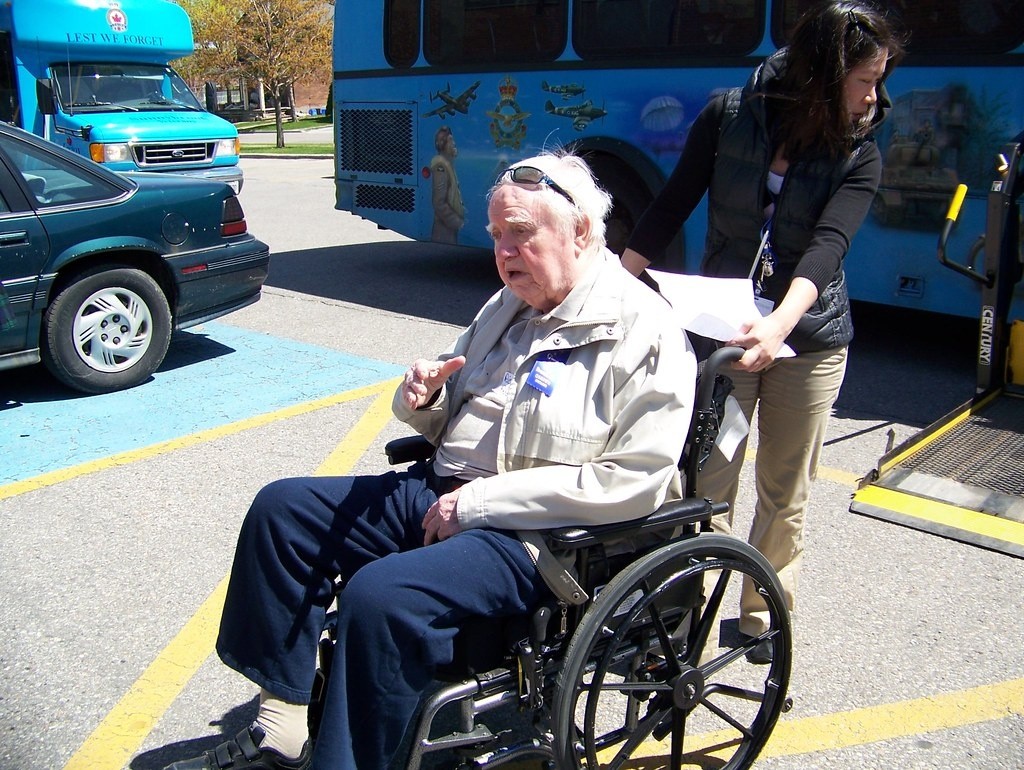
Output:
[740,628,774,664]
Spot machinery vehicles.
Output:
[852,136,1024,564]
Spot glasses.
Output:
[493,166,576,207]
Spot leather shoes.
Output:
[160,719,314,770]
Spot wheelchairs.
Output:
[310,346,794,769]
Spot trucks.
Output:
[0,0,246,197]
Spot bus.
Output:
[330,0,1024,366]
[0,119,270,397]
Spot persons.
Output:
[430,126,466,246]
[618,0,908,663]
[160,150,697,770]
